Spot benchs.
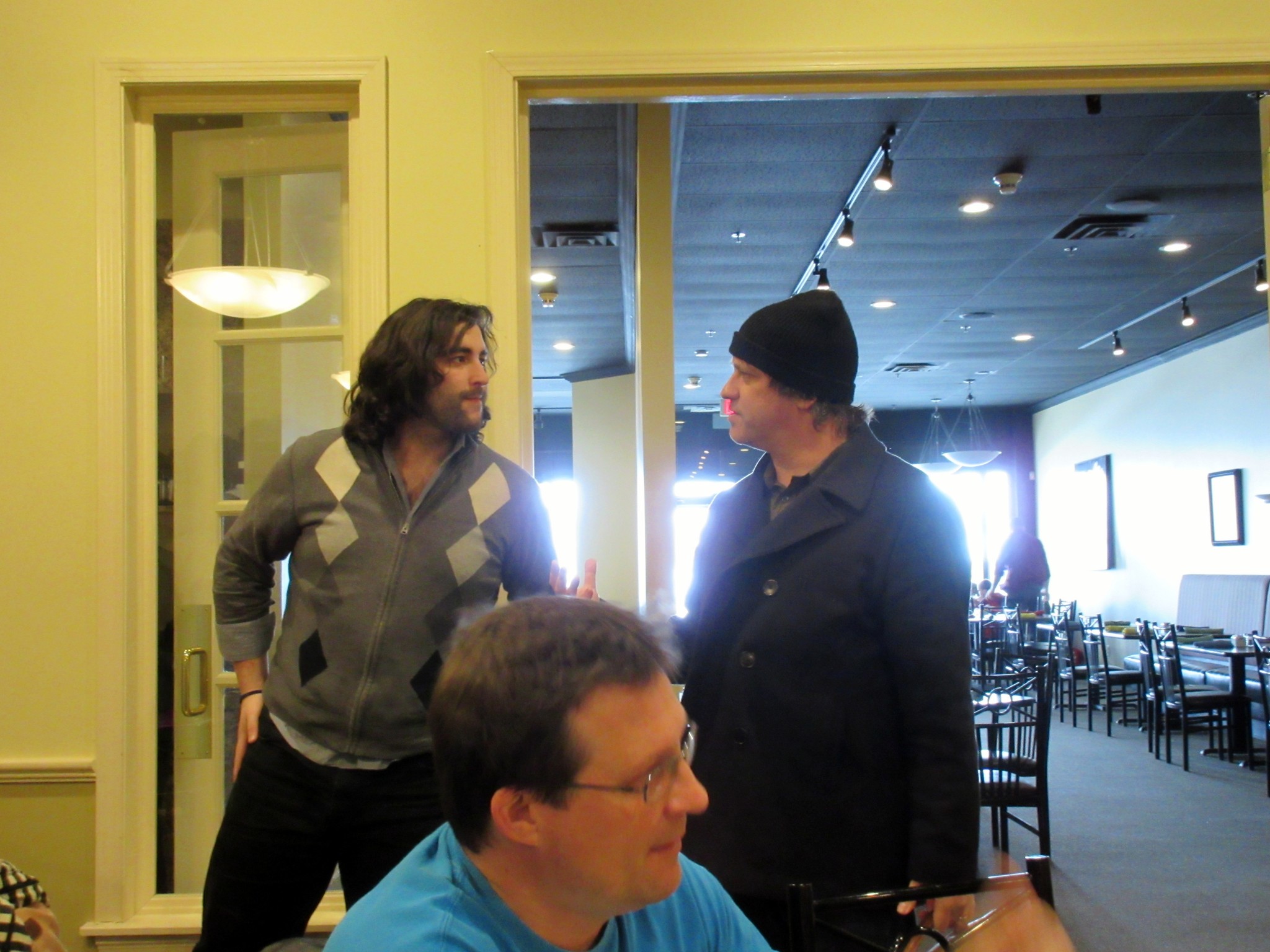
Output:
[1124,573,1270,740]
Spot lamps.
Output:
[160,174,332,321]
[1179,298,1196,327]
[873,144,896,192]
[836,206,855,248]
[1110,330,1125,357]
[812,257,830,291]
[910,399,959,474]
[1252,258,1270,291]
[941,382,1001,468]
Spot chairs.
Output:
[969,592,1270,859]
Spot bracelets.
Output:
[240,689,262,703]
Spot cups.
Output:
[1231,636,1246,647]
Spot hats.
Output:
[727,289,859,405]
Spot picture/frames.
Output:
[1206,467,1245,546]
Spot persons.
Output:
[985,517,1050,642]
[0,859,65,952]
[190,298,556,952]
[972,579,1005,614]
[547,292,980,952]
[319,598,778,952]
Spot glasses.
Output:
[566,719,699,804]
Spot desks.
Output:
[1087,626,1236,732]
[1161,640,1270,757]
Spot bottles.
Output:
[1243,633,1255,647]
